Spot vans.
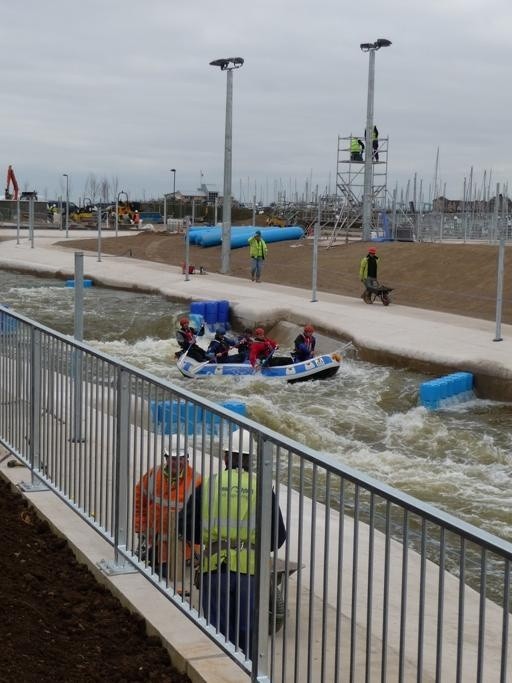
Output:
[47,200,82,214]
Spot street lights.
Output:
[208,52,244,280]
[355,35,394,239]
[169,168,177,219]
[61,173,70,238]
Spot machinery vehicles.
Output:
[4,164,39,202]
[263,207,311,242]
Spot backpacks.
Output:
[136,542,158,569]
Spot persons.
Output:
[48,203,57,223]
[176,317,207,362]
[293,325,315,363]
[358,246,382,299]
[349,137,364,162]
[248,231,268,283]
[364,125,381,161]
[133,209,140,224]
[178,428,286,660]
[204,327,293,371]
[134,433,201,578]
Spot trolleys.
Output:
[358,277,396,306]
[170,524,308,638]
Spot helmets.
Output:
[255,328,265,334]
[180,318,189,325]
[163,432,188,457]
[255,230,261,236]
[222,428,256,456]
[368,247,377,254]
[216,327,226,335]
[243,328,252,335]
[304,326,314,333]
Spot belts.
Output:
[203,538,257,557]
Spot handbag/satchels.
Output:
[194,563,203,591]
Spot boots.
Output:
[251,272,261,283]
[361,289,372,304]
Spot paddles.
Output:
[190,341,244,375]
[177,325,200,370]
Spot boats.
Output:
[165,338,347,386]
